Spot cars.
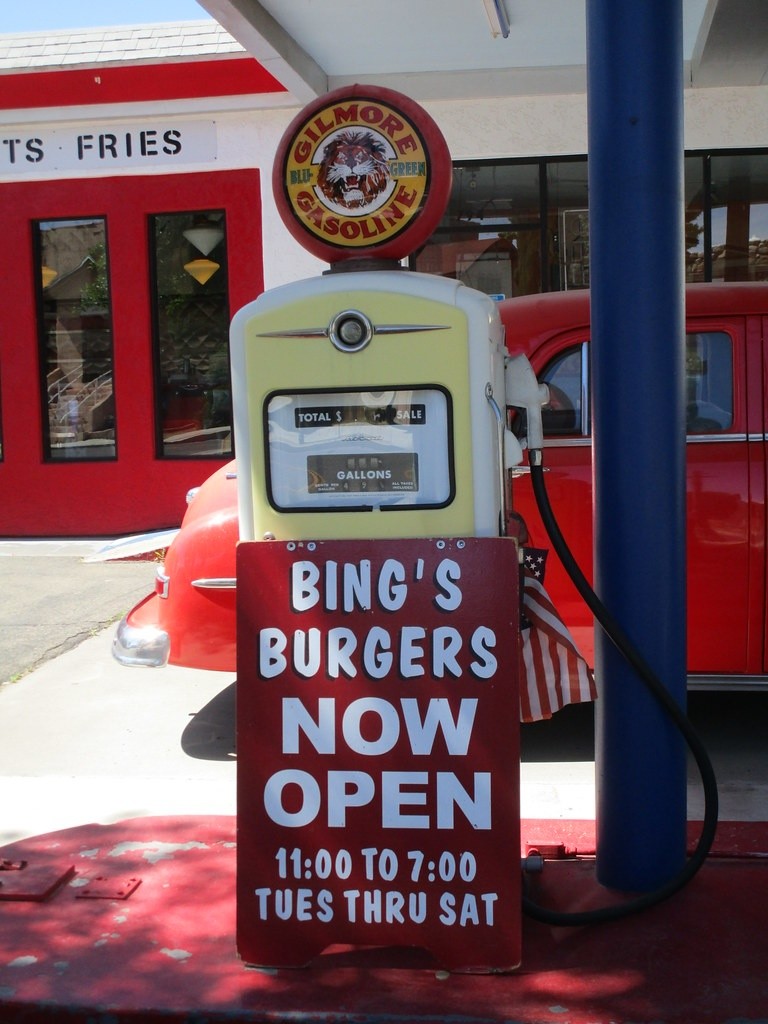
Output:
[112,282,768,755]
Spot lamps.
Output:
[183,214,224,257]
[184,251,220,285]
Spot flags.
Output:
[519,549,597,721]
